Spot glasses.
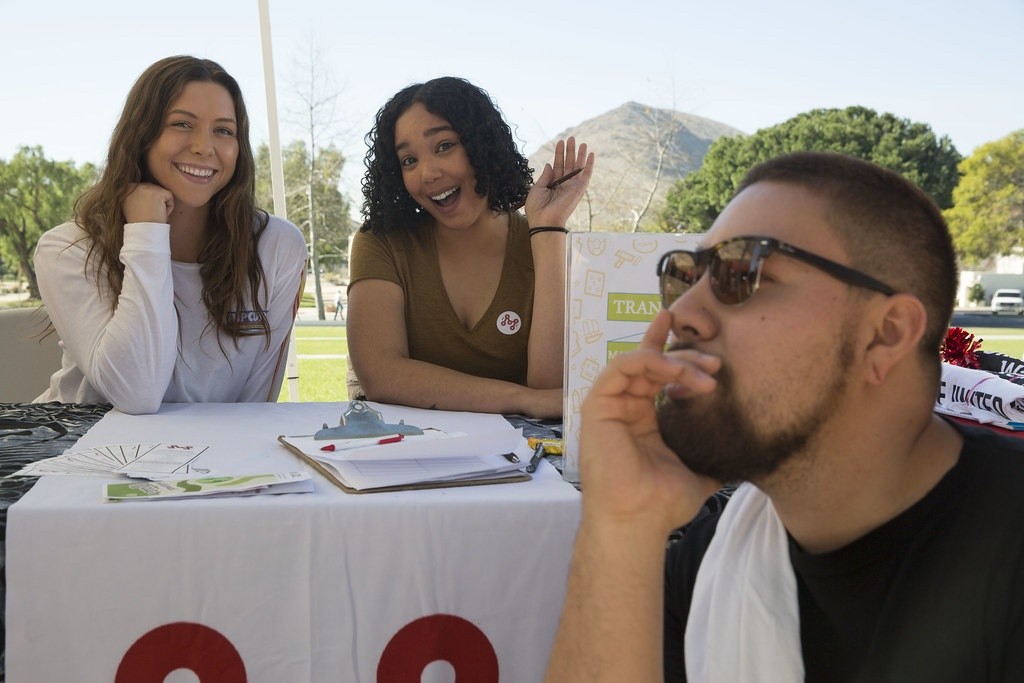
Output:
[656,234,899,307]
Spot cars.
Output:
[991,288,1023,315]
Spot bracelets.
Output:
[528,225,568,238]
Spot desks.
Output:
[0,402,588,683]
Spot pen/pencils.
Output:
[527,442,546,473]
[513,166,584,210]
[319,432,406,452]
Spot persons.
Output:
[539,150,1024,682]
[31,54,308,417]
[346,76,596,422]
